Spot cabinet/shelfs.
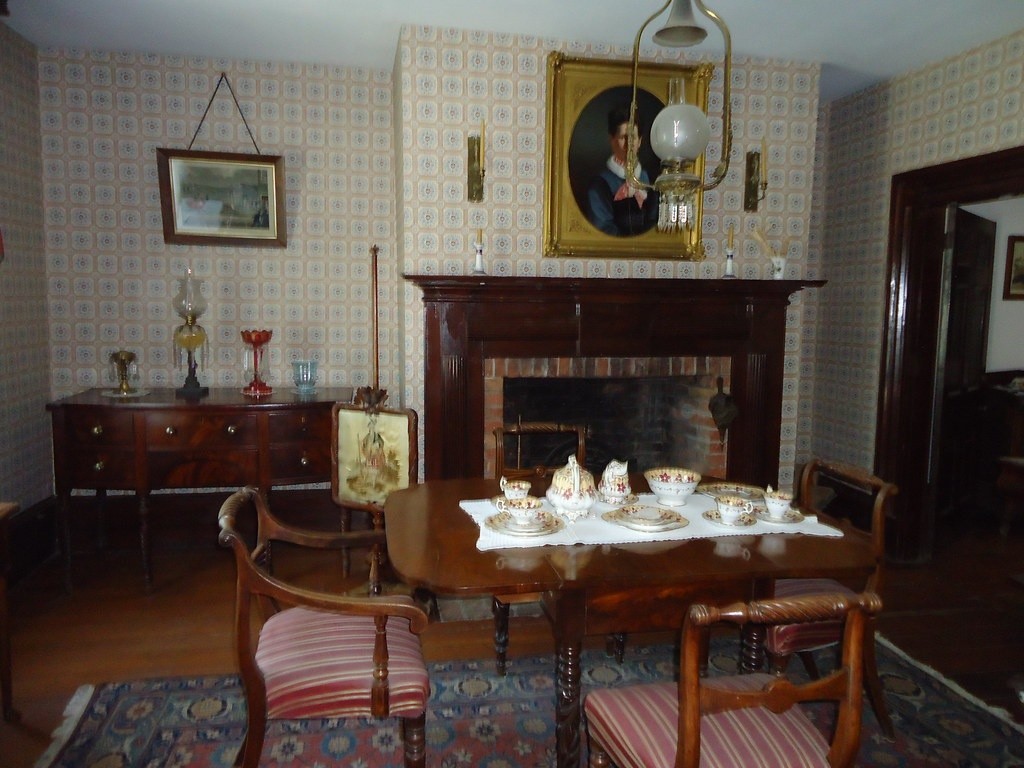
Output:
[46,384,355,514]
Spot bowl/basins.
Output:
[644,466,701,507]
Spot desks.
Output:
[384,476,878,768]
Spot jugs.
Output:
[598,459,632,506]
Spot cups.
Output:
[499,476,531,506]
[762,492,793,519]
[713,495,753,525]
[290,360,319,395]
[496,496,543,525]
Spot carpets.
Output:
[39,635,1024,768]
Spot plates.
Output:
[491,495,540,513]
[753,505,804,524]
[614,507,678,526]
[601,504,689,533]
[695,483,766,506]
[494,511,553,532]
[702,510,757,528]
[485,512,565,537]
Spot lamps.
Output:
[626,0,733,234]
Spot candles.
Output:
[726,224,735,249]
[477,229,482,244]
[759,135,768,180]
[480,120,484,169]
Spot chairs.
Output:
[218,483,431,768]
[493,424,626,675]
[585,590,882,768]
[771,459,900,736]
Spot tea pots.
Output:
[546,453,598,525]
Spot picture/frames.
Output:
[1001,235,1024,301]
[543,50,715,261]
[332,404,418,515]
[155,147,287,249]
